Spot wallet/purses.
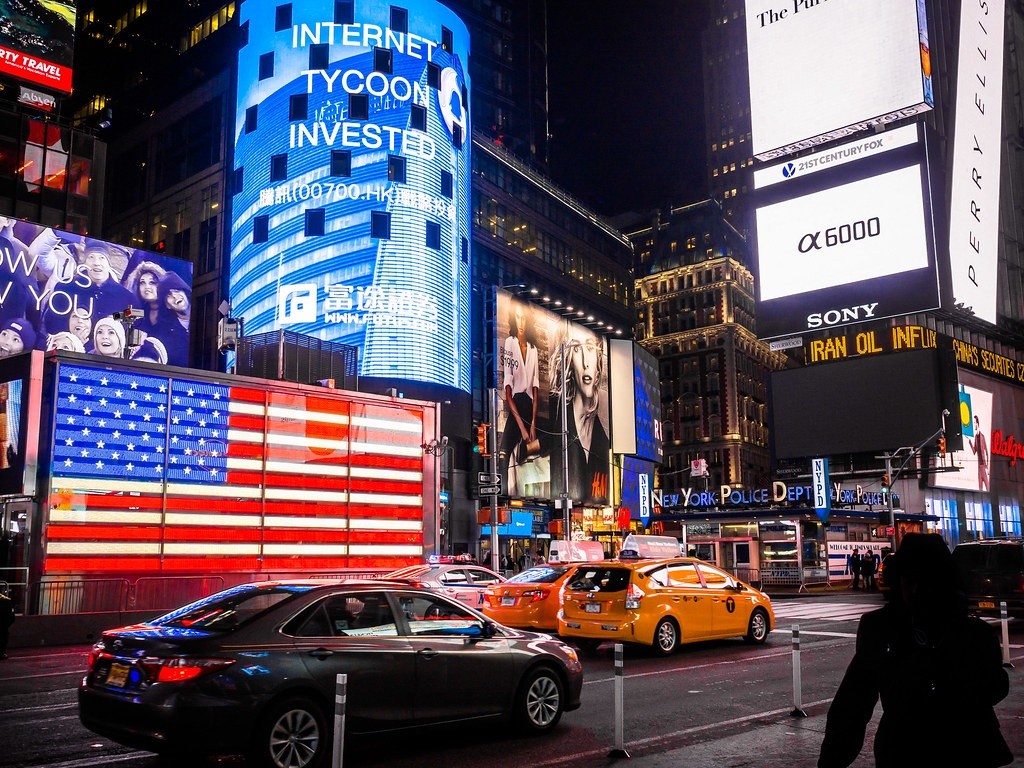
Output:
[526,439,540,453]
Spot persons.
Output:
[817,532,1014,768]
[499,296,610,500]
[0,216,193,367]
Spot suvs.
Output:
[952,535,1024,632]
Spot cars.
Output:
[479,541,608,635]
[77,579,585,768]
[554,534,775,658]
[377,554,509,612]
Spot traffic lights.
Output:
[472,423,487,456]
[498,507,513,525]
[936,435,947,458]
[880,473,890,493]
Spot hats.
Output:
[0,317,36,350]
[69,305,93,322]
[84,240,111,266]
[93,315,125,359]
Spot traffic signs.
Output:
[477,473,503,485]
[478,485,504,497]
